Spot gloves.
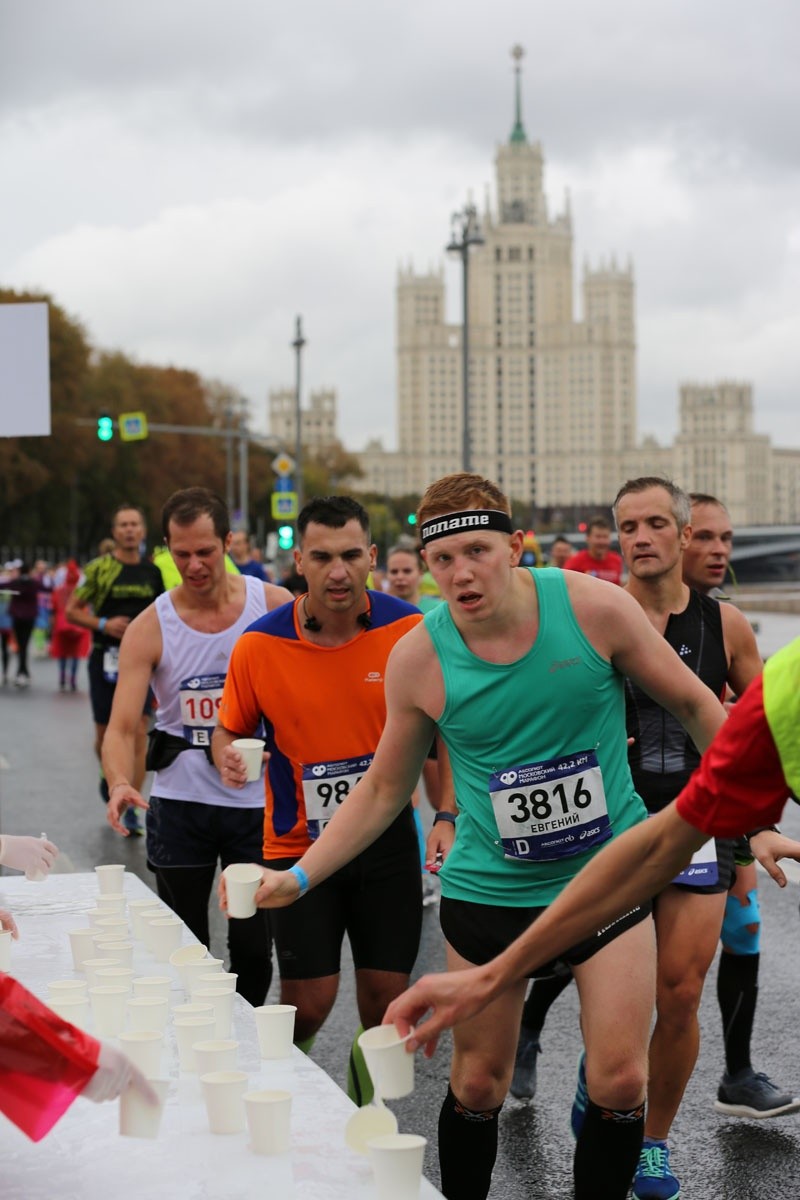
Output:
[0,833,60,873]
[77,1043,161,1109]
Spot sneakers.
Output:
[712,1071,800,1119]
[571,1052,591,1140]
[631,1142,680,1200]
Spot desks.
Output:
[0,871,449,1200]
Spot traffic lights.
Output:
[97,417,115,442]
[279,526,298,551]
[408,512,417,526]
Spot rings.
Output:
[224,767,228,776]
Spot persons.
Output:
[218,475,800,1200]
[0,501,626,837]
[99,491,296,1008]
[380,635,799,1058]
[614,474,798,1200]
[507,494,800,1119]
[0,835,58,940]
[209,496,458,1110]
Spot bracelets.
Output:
[433,812,457,826]
[110,782,128,796]
[98,617,107,632]
[743,826,780,842]
[289,865,309,900]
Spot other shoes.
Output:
[100,776,111,803]
[510,999,546,1102]
[122,812,145,836]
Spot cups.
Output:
[230,738,266,783]
[0,862,432,1200]
